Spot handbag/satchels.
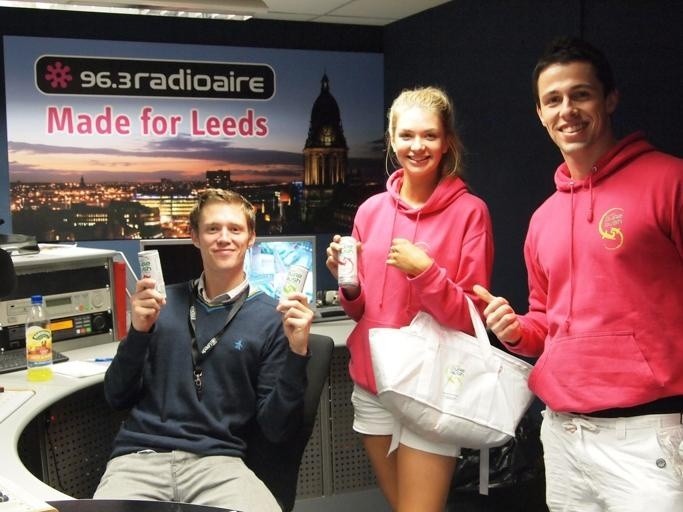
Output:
[367,294,538,451]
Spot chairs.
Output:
[244,332,336,512]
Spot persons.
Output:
[320,83,499,511]
[472,39,683,512]
[91,188,315,511]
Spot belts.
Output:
[570,395,683,420]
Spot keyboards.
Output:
[0,346,69,374]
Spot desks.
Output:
[0,315,376,511]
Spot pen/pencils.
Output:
[87,357,115,362]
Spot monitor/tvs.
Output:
[140,239,203,285]
[242,234,317,308]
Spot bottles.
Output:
[24,295,55,384]
